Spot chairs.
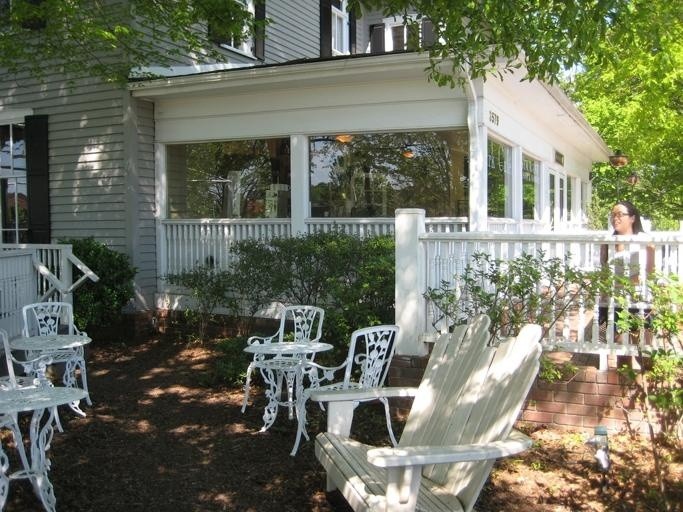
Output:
[241,305,401,456]
[1,300,94,512]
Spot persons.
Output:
[597,200,656,372]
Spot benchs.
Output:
[310,312,543,512]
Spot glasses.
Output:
[607,210,632,219]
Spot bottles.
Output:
[593,425,612,474]
